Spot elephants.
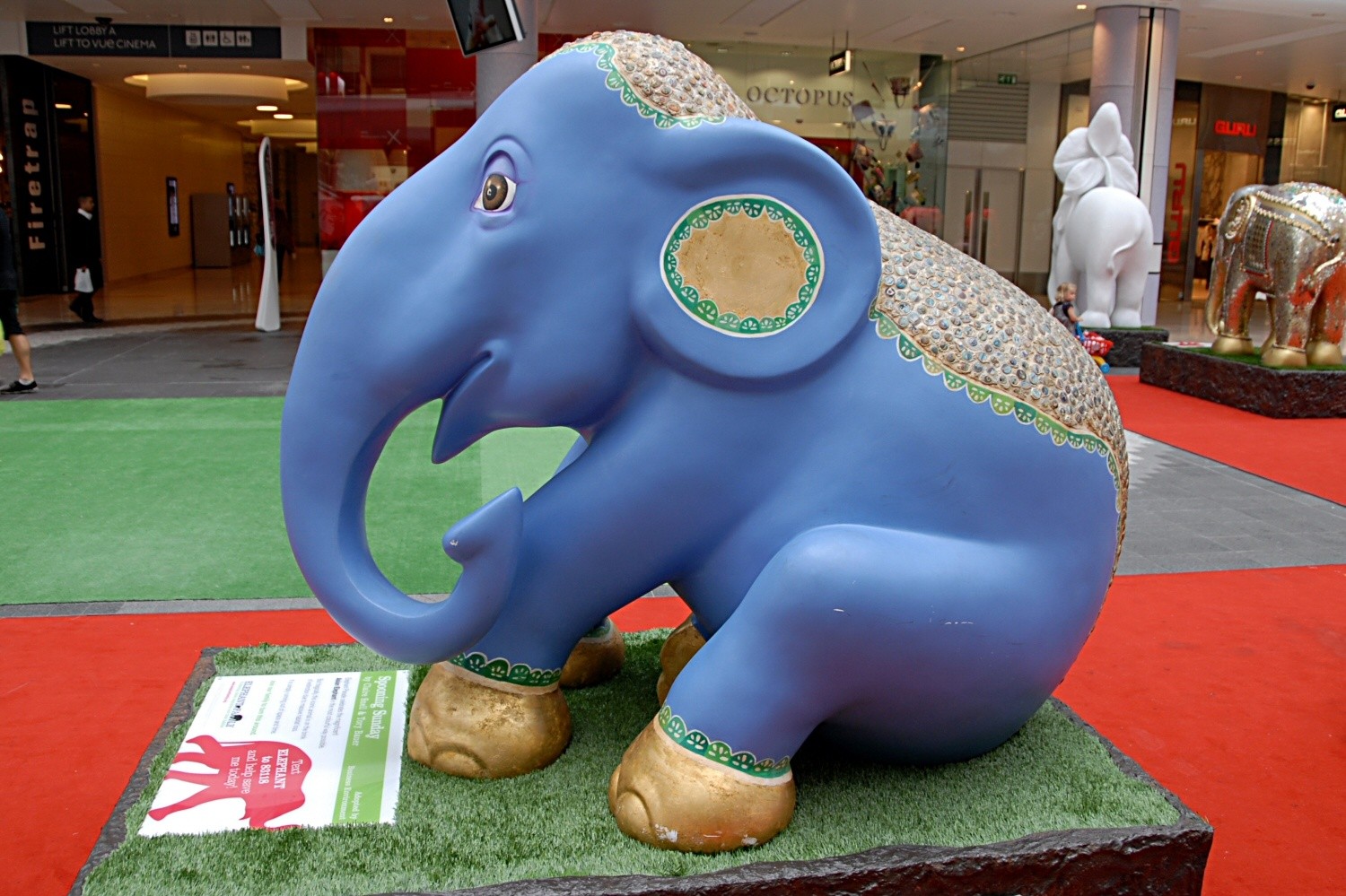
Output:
[1204,178,1346,371]
[278,26,1129,854]
[1048,186,1154,328]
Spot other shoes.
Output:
[0,380,38,393]
[82,316,102,324]
[68,303,85,318]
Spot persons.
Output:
[1048,282,1083,338]
[0,192,38,394]
[68,192,104,325]
